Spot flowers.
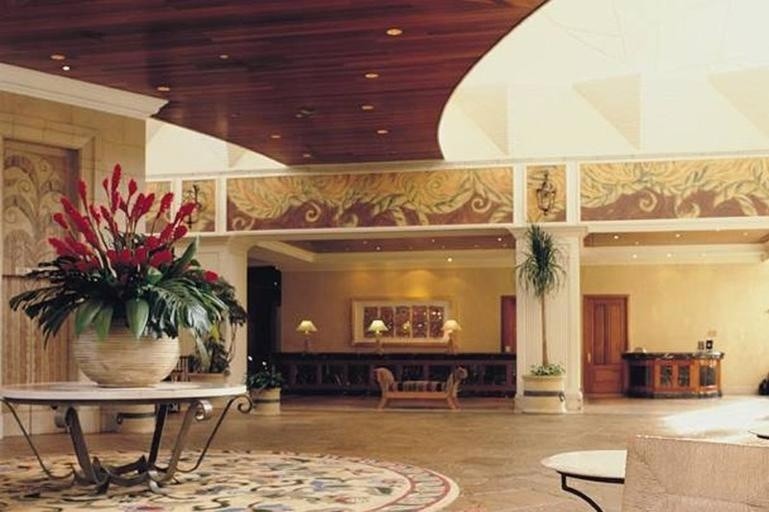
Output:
[3,161,250,353]
[244,354,291,394]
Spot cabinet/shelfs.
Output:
[346,297,452,347]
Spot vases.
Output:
[250,387,282,414]
[71,311,182,391]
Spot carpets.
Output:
[2,447,462,510]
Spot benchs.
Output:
[372,367,468,411]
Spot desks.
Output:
[2,378,259,503]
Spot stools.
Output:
[539,446,629,512]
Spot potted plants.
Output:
[512,219,574,415]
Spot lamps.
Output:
[535,171,558,217]
[366,317,389,353]
[440,318,462,349]
[296,318,318,350]
